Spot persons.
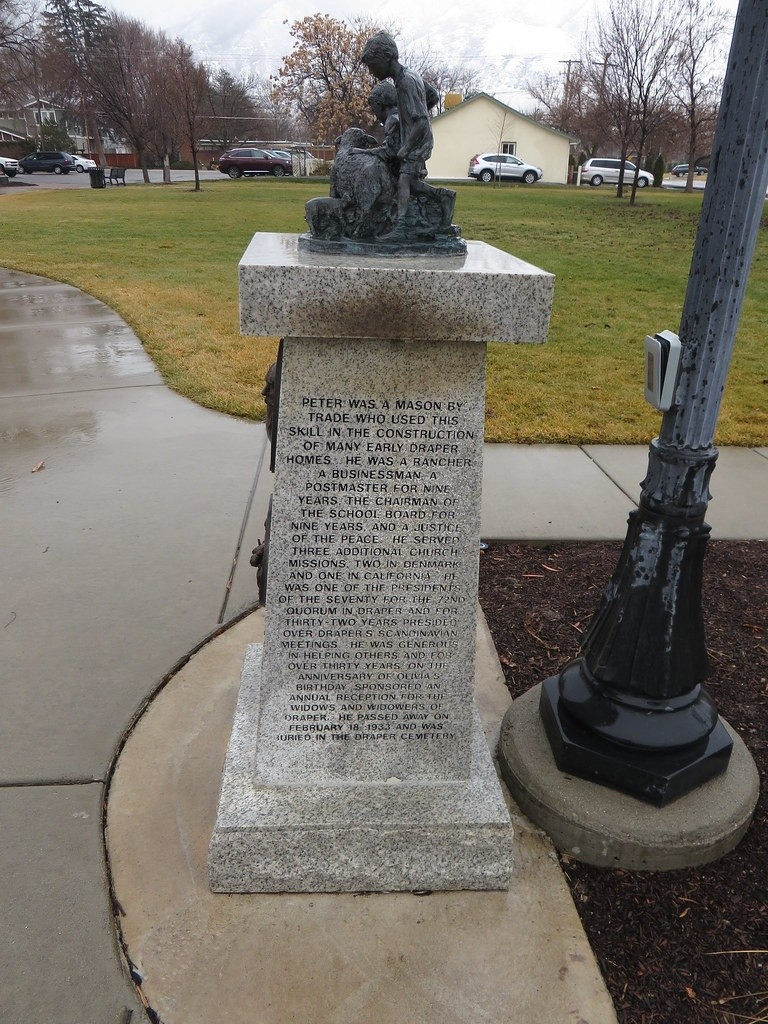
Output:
[349,29,457,240]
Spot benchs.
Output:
[103,168,126,186]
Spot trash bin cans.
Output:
[88,167,106,189]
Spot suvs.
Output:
[468,153,543,184]
[219,148,315,178]
[581,158,654,188]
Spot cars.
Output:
[671,164,708,177]
[71,155,96,173]
[0,157,18,177]
[18,151,75,174]
